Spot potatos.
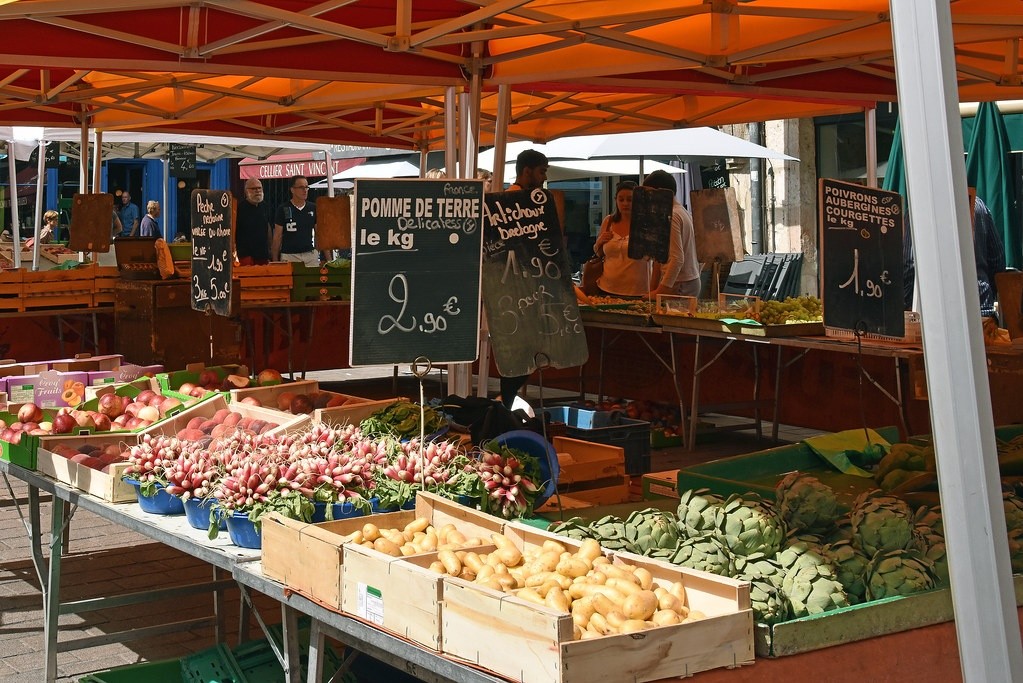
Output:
[341,518,708,642]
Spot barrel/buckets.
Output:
[167,242,192,261]
[174,261,192,278]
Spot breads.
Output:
[154,238,175,279]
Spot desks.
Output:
[581,321,764,449]
[662,325,921,453]
[231,496,1023,682]
[0,459,259,683]
[242,301,349,383]
[0,306,112,355]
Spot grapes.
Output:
[750,293,823,326]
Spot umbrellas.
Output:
[883,101,1020,271]
[307,126,801,182]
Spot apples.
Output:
[582,396,686,437]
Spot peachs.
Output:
[0,369,364,468]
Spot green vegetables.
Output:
[356,401,461,441]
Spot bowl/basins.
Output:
[124,421,559,551]
[343,646,420,683]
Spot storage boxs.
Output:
[0,243,1023,683]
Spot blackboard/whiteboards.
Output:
[349,178,485,367]
[627,187,674,263]
[699,159,730,188]
[482,188,589,377]
[190,188,233,317]
[169,143,196,179]
[819,176,905,339]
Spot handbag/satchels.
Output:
[578,256,603,296]
[25,237,35,247]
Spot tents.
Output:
[0,0,1023,683]
[0,126,334,271]
[237,153,368,179]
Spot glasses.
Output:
[294,186,310,191]
[246,186,262,190]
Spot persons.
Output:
[500,149,549,411]
[594,181,653,296]
[271,175,332,261]
[40,210,59,244]
[477,168,493,193]
[140,200,161,236]
[643,170,702,297]
[1,217,25,242]
[112,191,139,235]
[427,168,446,179]
[236,179,272,266]
[903,197,1006,327]
